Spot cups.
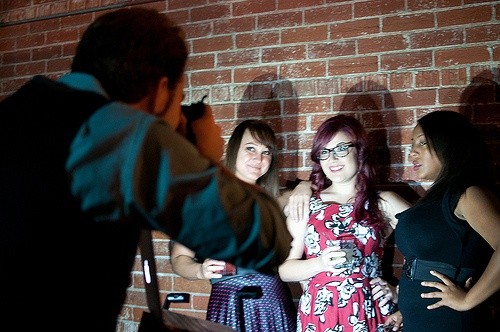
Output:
[213,261,236,276]
[330,239,355,269]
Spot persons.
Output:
[169,120,319,332]
[383,110,500,332]
[0,7,293,332]
[277,115,413,332]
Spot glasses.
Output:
[316,140,357,160]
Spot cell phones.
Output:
[377,325,404,332]
